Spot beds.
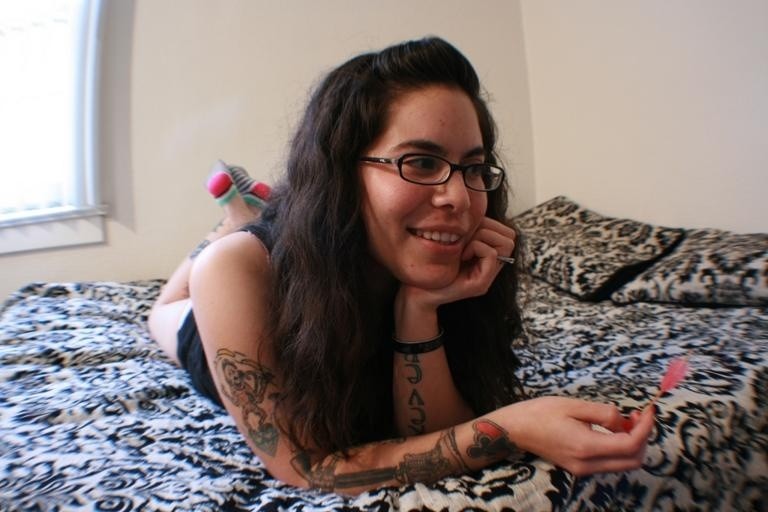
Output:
[0,212,766,512]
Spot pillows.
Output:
[611,225,768,305]
[508,193,690,305]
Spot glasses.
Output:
[357,152,507,193]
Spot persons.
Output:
[143,37,658,495]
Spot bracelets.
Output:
[391,329,445,354]
